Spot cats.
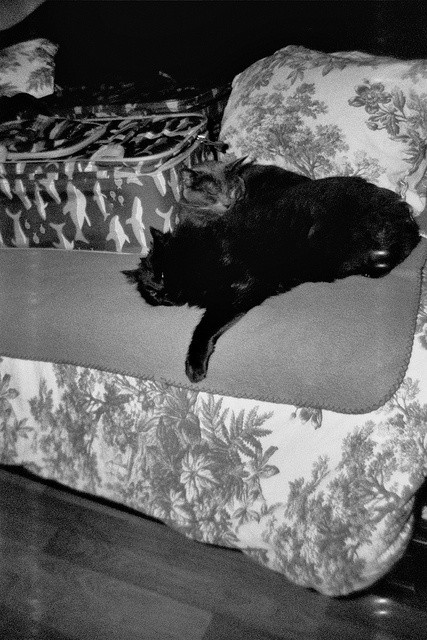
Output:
[176,155,248,227]
[120,175,419,384]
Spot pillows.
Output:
[0,39,59,98]
[219,44,427,215]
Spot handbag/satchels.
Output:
[70,69,232,134]
[0,112,213,254]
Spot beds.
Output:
[1,0,425,597]
